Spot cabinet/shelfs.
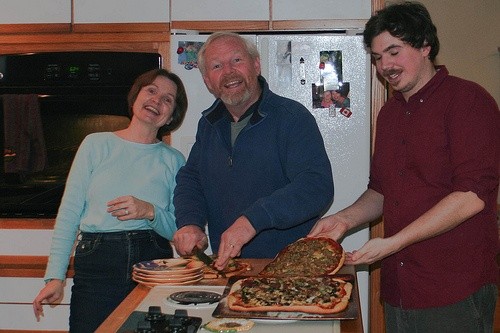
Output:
[0,0,371,34]
[0,218,82,333]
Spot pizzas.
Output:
[256,237,345,277]
[228,276,353,314]
[204,256,243,274]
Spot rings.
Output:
[125,209,128,215]
[229,244,234,248]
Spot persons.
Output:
[308,2,500,333]
[172,33,335,271]
[317,87,350,107]
[32,69,188,333]
